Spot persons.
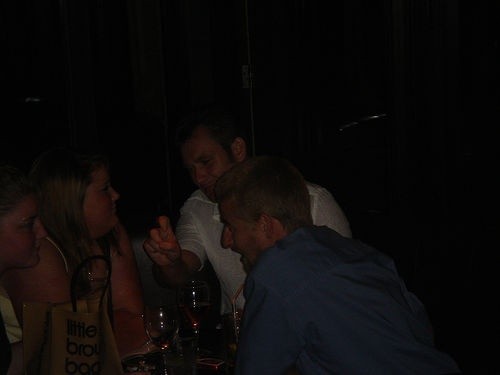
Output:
[0,165,48,375]
[142,111,353,318]
[26,153,128,375]
[212,154,466,375]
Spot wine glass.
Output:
[176,280,211,359]
[143,303,180,375]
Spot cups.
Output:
[224,302,243,368]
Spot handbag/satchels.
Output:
[39,254,127,374]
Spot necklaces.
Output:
[86,269,96,280]
